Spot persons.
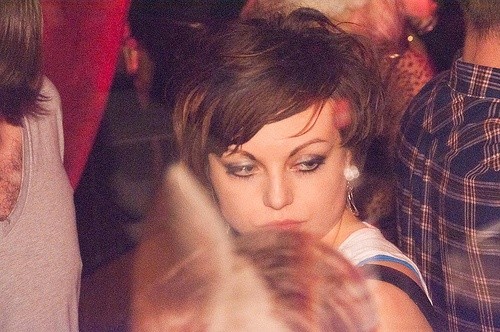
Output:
[0,0,84,332]
[129,229,377,332]
[171,0,500,332]
